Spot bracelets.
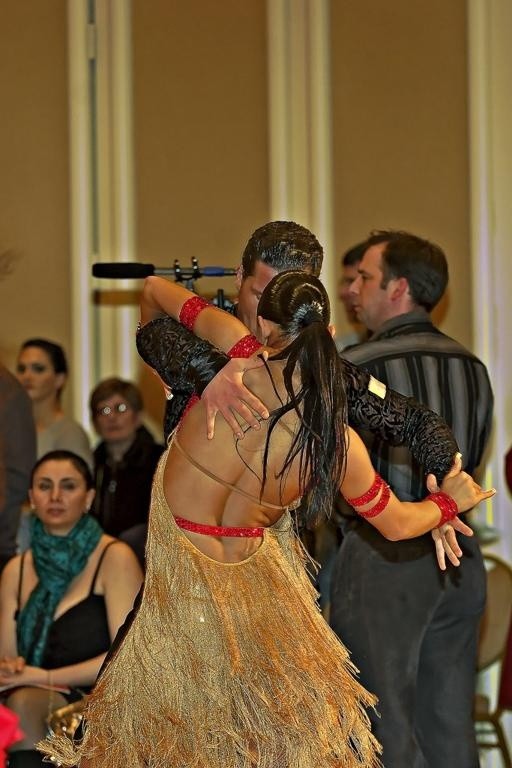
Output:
[423,492,459,530]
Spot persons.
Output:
[88,378,169,577]
[134,220,478,573]
[0,450,146,767]
[305,226,495,767]
[16,336,95,485]
[323,240,376,353]
[38,269,499,767]
[0,364,40,572]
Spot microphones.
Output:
[91,260,240,282]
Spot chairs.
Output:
[469,553,511,768]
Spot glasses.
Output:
[89,404,134,417]
